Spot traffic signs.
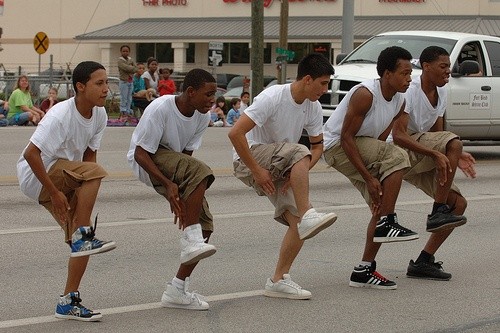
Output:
[277,56,294,62]
[276,47,295,56]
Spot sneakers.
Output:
[160,283,209,311]
[373,212,419,242]
[348,260,397,290]
[425,205,467,232]
[263,274,312,299]
[178,223,217,264]
[55,290,103,322]
[297,208,339,240]
[405,258,452,281]
[70,225,116,257]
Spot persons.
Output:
[126,68,220,311]
[229,54,338,300]
[117,45,176,122]
[40,88,61,114]
[16,60,116,324]
[384,46,479,283]
[208,92,251,126]
[317,45,421,290]
[6,76,45,125]
[0,99,9,119]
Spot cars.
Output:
[211,76,295,105]
[314,28,499,146]
